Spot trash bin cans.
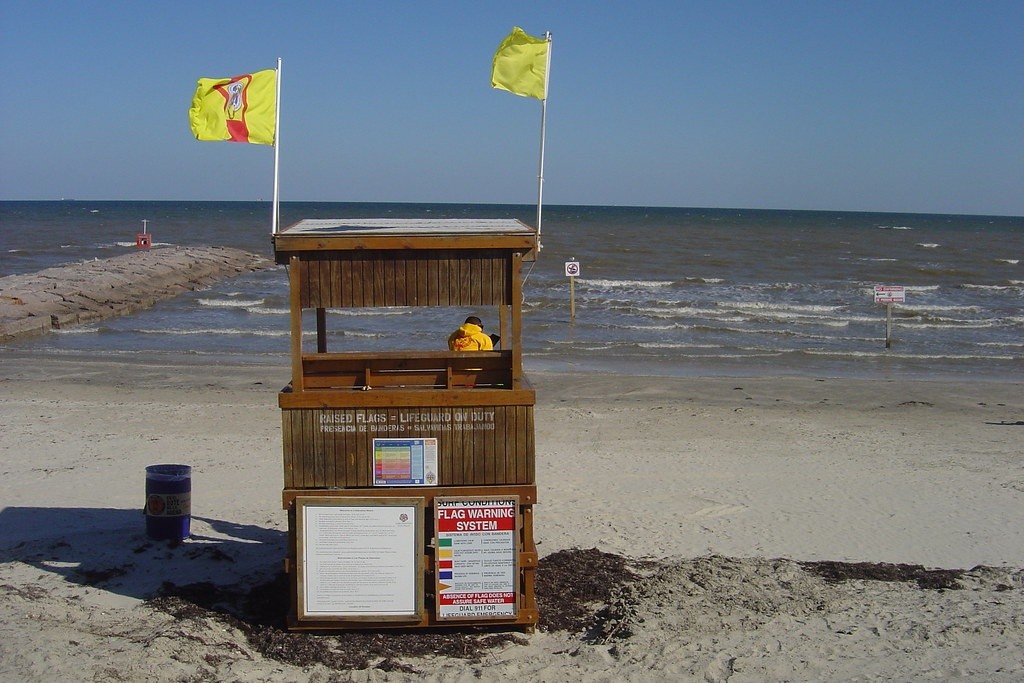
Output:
[144,464,192,539]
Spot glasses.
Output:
[479,324,484,328]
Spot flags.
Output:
[189,68,277,146]
[492,26,551,100]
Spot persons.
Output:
[448,316,493,387]
